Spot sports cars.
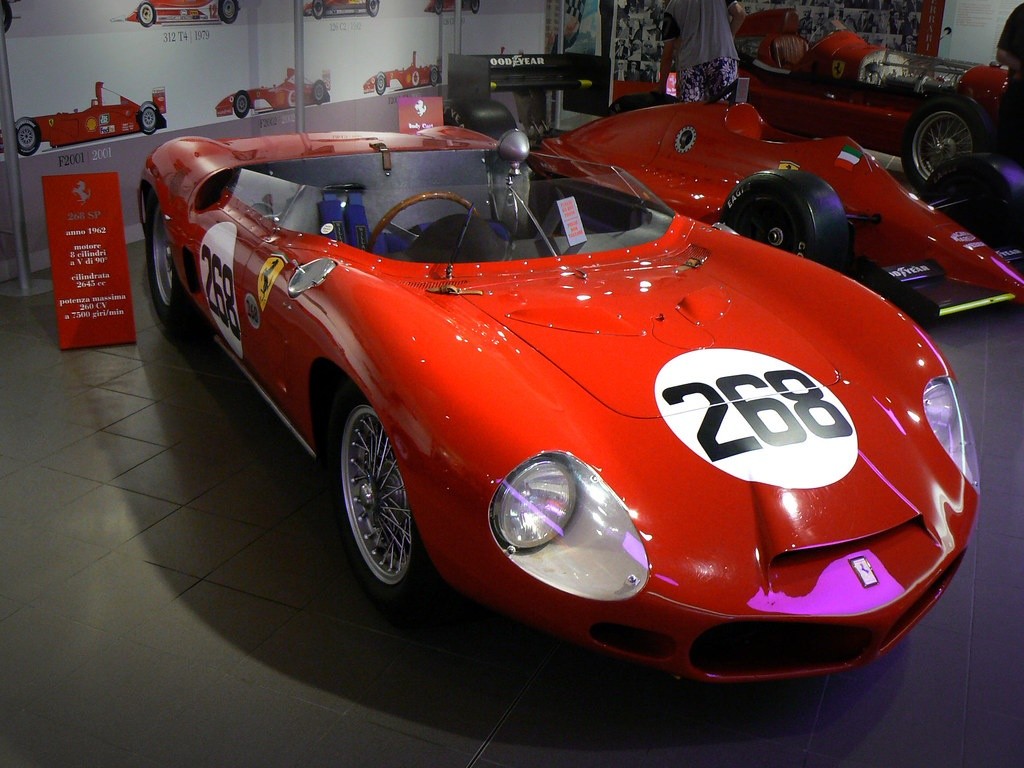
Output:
[138,125,978,681]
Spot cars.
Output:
[613,7,1008,192]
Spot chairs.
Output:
[772,11,811,70]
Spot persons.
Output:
[658,0,746,105]
[995,3,1024,170]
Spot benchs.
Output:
[231,143,568,238]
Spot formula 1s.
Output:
[443,92,1024,322]
